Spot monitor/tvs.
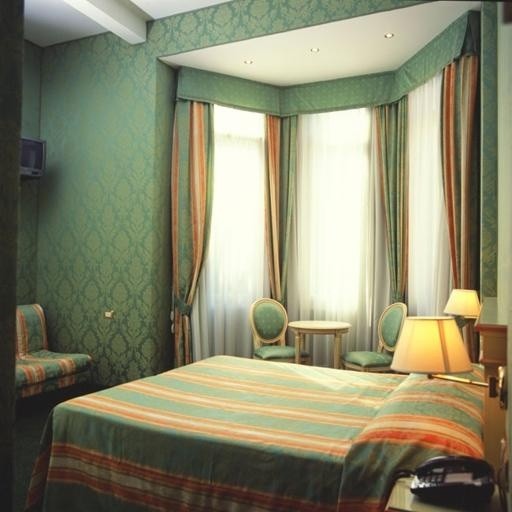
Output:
[20,136,46,177]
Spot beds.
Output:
[52,324,511,512]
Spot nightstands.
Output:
[386,472,505,512]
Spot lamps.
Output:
[443,289,481,320]
[389,316,508,410]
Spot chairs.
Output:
[340,301,407,372]
[249,297,312,365]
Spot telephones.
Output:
[410,456,495,512]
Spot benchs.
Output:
[15,302,93,399]
